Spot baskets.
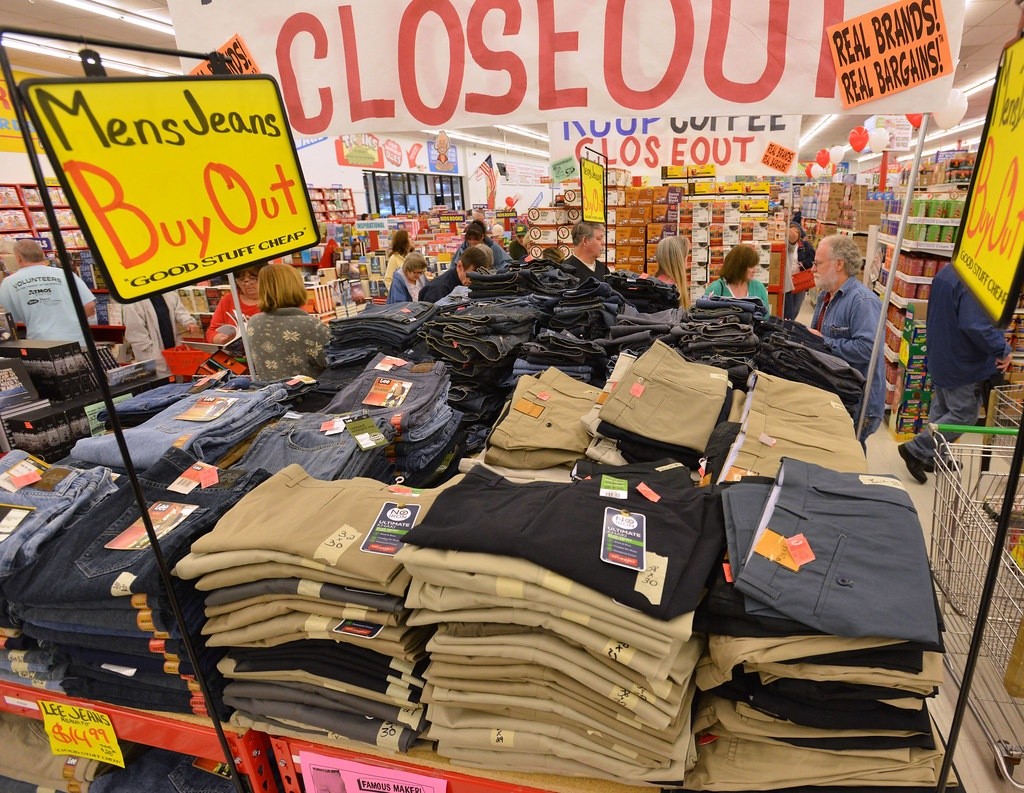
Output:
[792,262,815,294]
[161,330,215,375]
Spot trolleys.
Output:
[925,419,1024,792]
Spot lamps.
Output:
[0,0,179,78]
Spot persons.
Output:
[382,223,563,305]
[808,234,886,461]
[361,213,370,220]
[898,243,1013,484]
[204,263,269,345]
[656,235,691,308]
[783,222,815,323]
[705,245,768,320]
[0,241,97,346]
[125,291,201,372]
[559,220,609,280]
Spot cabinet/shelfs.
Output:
[15,290,127,344]
[872,213,961,414]
[306,186,357,222]
[0,183,89,255]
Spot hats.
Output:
[515,224,526,236]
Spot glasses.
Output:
[234,277,257,286]
[411,269,424,275]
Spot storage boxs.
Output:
[311,218,466,319]
[528,181,884,318]
[920,174,932,187]
[886,301,1024,444]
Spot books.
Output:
[0,186,125,453]
[178,190,473,337]
[179,310,251,358]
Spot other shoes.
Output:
[924,459,963,472]
[898,443,927,483]
